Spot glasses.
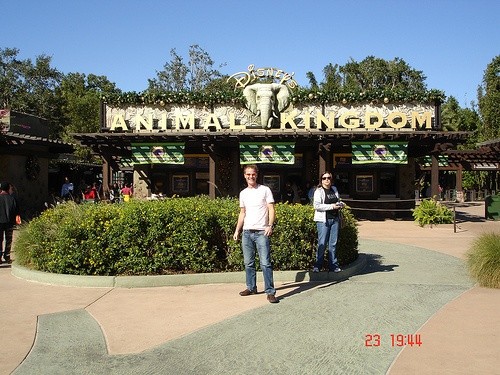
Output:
[321,177,331,180]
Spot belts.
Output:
[249,230,259,233]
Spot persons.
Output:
[0,181,21,264]
[151,190,167,199]
[312,171,346,273]
[233,165,277,303]
[74,180,133,202]
[61,176,73,200]
[281,183,317,205]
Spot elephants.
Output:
[243,83,291,129]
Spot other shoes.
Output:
[313,266,322,272]
[267,294,279,303]
[239,289,257,296]
[330,266,341,273]
